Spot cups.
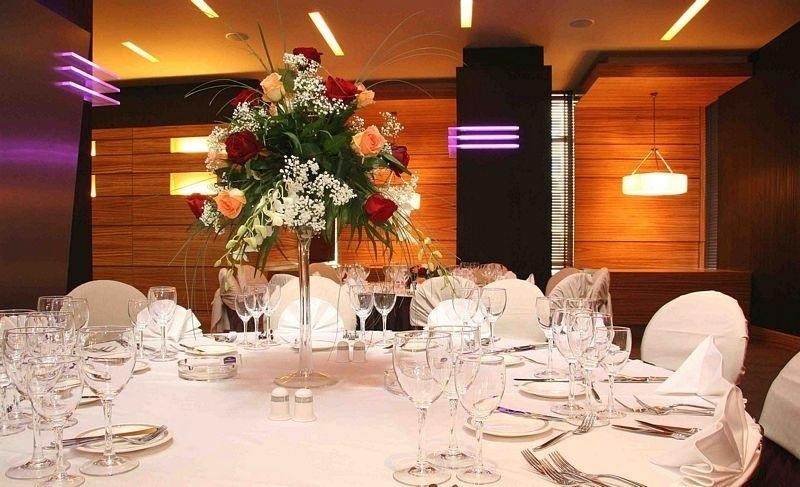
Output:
[334,341,353,363]
[267,386,292,422]
[293,387,316,423]
[353,340,367,363]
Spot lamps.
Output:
[621,92,688,197]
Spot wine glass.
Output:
[1,285,180,487]
[335,261,503,292]
[391,319,453,486]
[348,284,397,349]
[454,355,507,485]
[234,285,283,351]
[425,325,481,468]
[451,288,507,355]
[534,297,632,427]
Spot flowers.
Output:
[165,0,463,335]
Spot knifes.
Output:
[51,427,160,445]
[602,375,668,382]
[613,418,702,440]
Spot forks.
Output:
[615,393,716,418]
[41,425,168,451]
[520,447,646,486]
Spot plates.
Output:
[462,410,551,436]
[182,344,235,355]
[506,355,525,365]
[72,422,172,449]
[74,383,101,407]
[290,339,336,351]
[132,360,152,374]
[518,379,588,399]
